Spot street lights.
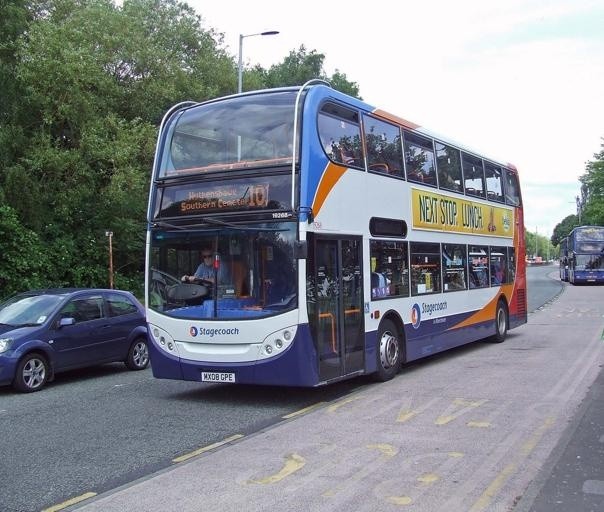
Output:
[236,30,283,162]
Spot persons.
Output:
[178,245,227,289]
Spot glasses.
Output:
[201,254,211,259]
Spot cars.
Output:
[0,288,148,393]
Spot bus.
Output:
[558,225,603,286]
[142,78,529,390]
